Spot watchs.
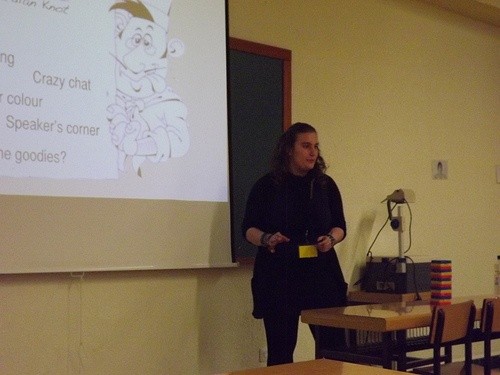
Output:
[326,233,336,249]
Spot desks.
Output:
[222,297,500,375]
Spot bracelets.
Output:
[260,231,273,248]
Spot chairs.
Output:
[413,297,476,375]
[471,298,500,375]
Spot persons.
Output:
[242,122,349,367]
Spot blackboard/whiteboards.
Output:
[227,36,294,264]
